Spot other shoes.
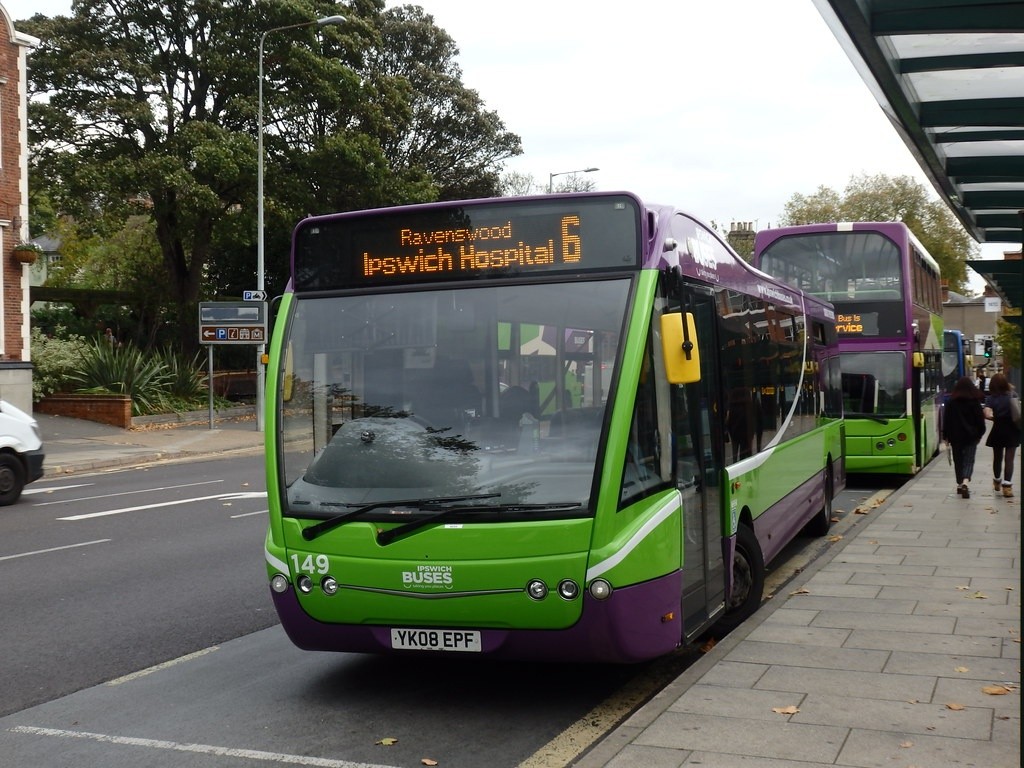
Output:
[1002,482,1014,497]
[957,485,962,494]
[962,484,969,498]
[993,479,1002,491]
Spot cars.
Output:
[0,399,46,505]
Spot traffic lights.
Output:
[984,337,993,359]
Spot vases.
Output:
[12,249,39,263]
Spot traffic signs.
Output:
[200,325,267,344]
[242,290,269,302]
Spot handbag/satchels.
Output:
[1010,391,1021,422]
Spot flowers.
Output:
[12,240,44,272]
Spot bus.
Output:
[755,220,945,482]
[253,191,846,666]
[941,328,974,405]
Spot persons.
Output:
[412,357,472,436]
[977,370,986,404]
[983,373,1023,497]
[942,377,986,498]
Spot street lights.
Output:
[549,168,600,193]
[255,15,348,435]
